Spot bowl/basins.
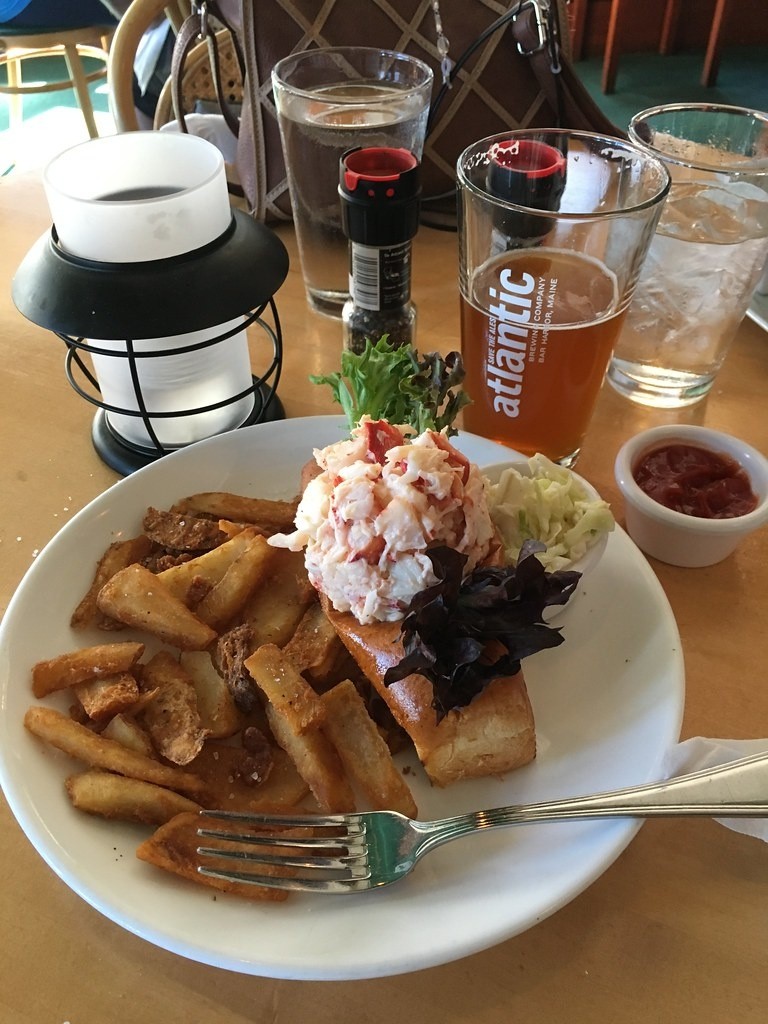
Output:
[613,422,768,568]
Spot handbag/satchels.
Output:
[172,0,632,231]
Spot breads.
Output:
[299,459,536,787]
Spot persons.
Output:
[0,0,176,131]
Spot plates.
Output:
[0,414,686,981]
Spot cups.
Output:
[271,45,436,319]
[604,102,768,408]
[456,127,672,475]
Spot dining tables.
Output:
[1,107,767,1024]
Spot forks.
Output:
[196,750,768,893]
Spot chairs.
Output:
[1,0,245,138]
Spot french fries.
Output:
[21,490,419,905]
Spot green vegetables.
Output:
[384,540,584,727]
[306,338,475,444]
